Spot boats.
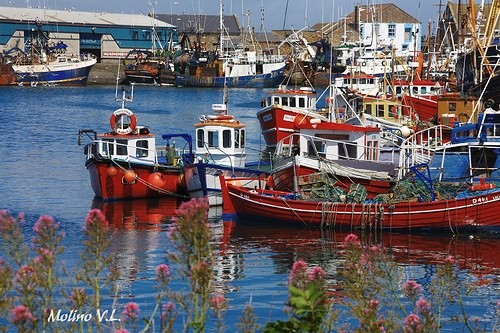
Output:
[261,0,500,157]
[226,177,499,230]
[182,90,268,205]
[77,58,182,201]
[270,134,401,199]
[115,0,262,89]
[0,0,98,87]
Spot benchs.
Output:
[451,112,500,143]
